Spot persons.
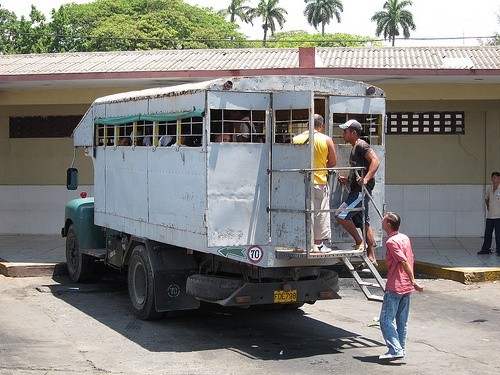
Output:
[291,114,337,253]
[477,172,500,257]
[118,110,294,147]
[334,119,380,269]
[378,212,423,359]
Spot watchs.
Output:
[411,281,416,286]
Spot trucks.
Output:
[60,75,388,321]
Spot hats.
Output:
[339,119,362,131]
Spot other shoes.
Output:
[477,250,491,254]
[496,251,500,256]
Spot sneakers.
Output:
[355,240,368,250]
[361,259,378,268]
[313,241,331,253]
[379,350,406,359]
